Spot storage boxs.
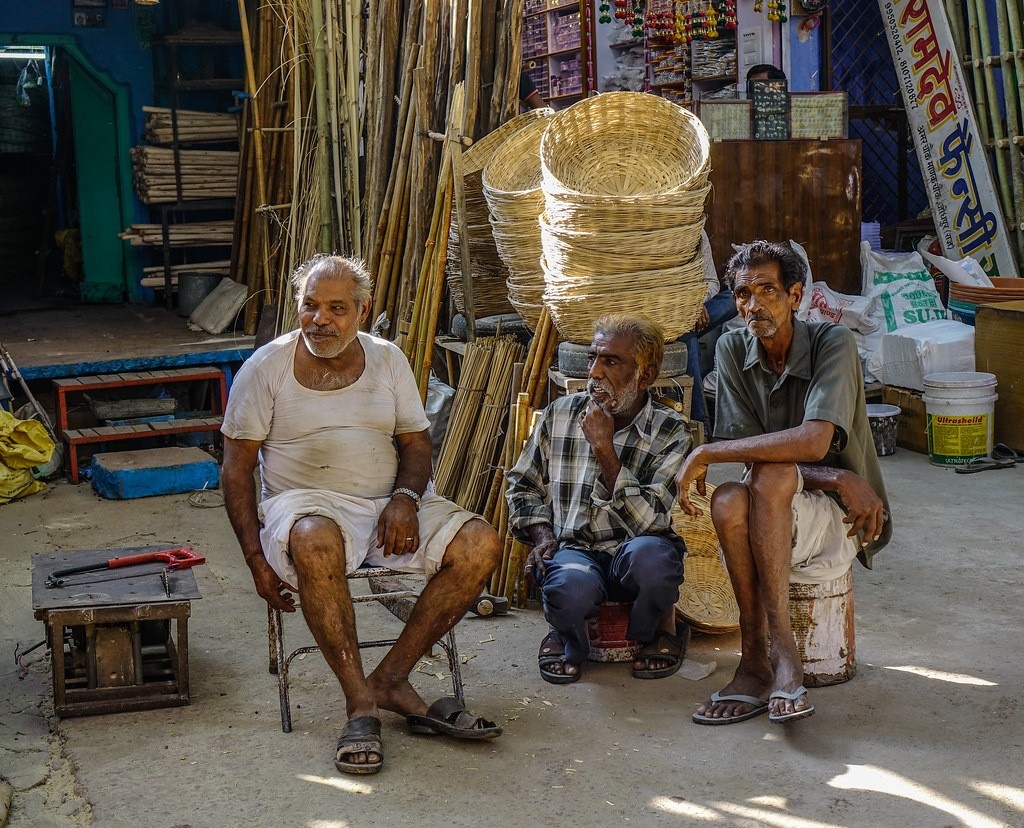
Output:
[882,385,928,454]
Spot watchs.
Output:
[391,488,422,512]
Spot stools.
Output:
[269,566,465,733]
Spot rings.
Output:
[406,537,413,541]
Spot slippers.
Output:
[334,716,384,773]
[991,443,1024,463]
[538,632,581,684]
[692,690,769,725]
[633,622,691,679]
[769,686,816,724]
[406,697,503,740]
[955,456,1016,474]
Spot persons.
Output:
[220,256,501,772]
[676,227,737,444]
[505,314,695,686]
[674,239,893,724]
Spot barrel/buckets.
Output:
[770,567,858,685]
[584,602,678,661]
[177,272,223,316]
[946,277,1024,326]
[919,373,999,467]
[866,403,902,456]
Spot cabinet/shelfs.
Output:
[155,76,245,313]
[517,0,738,115]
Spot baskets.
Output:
[674,478,741,634]
[446,90,713,346]
